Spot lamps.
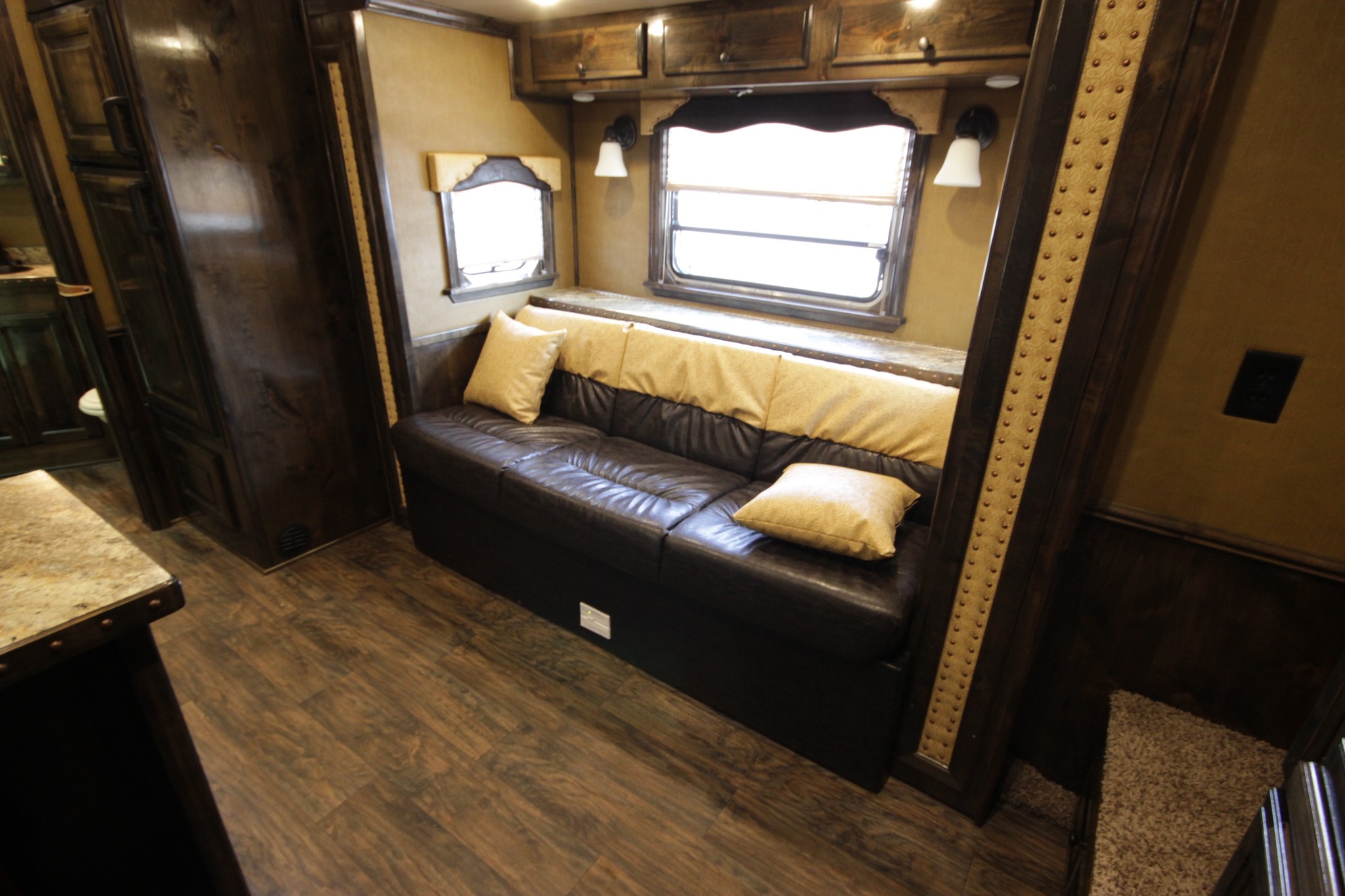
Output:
[934,103,1003,193]
[589,115,638,179]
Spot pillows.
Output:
[733,459,922,562]
[459,312,568,421]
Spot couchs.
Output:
[387,301,958,790]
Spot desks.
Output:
[2,467,256,894]
